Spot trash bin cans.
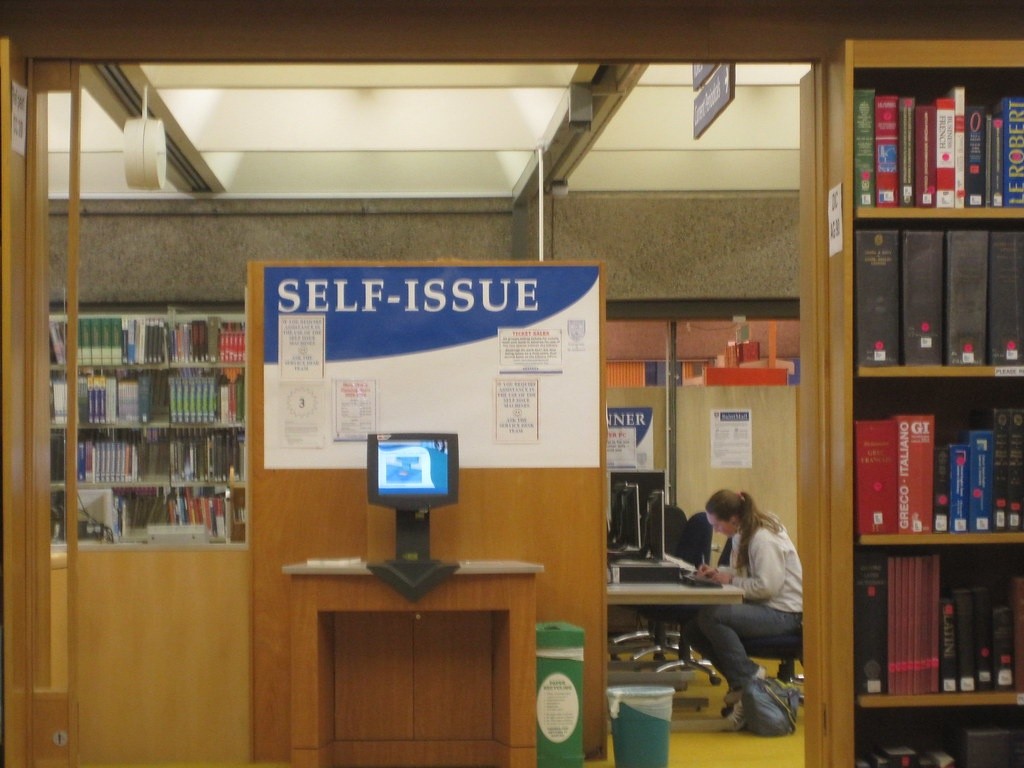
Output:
[606,684,673,768]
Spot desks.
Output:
[606,544,744,605]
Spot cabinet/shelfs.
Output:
[282,562,543,768]
[47,313,247,548]
[798,38,1024,767]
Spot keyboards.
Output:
[682,574,724,589]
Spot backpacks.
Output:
[742,677,798,737]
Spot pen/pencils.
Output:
[702,554,705,566]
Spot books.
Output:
[855,229,1024,365]
[854,720,1024,768]
[853,549,1024,693]
[854,86,1024,208]
[854,407,1024,534]
[77,317,245,535]
[50,321,67,543]
[607,341,761,388]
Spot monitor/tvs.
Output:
[367,432,458,511]
[616,483,666,563]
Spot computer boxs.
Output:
[609,558,681,583]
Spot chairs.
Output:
[609,505,804,717]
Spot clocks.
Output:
[122,86,167,192]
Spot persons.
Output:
[682,489,804,729]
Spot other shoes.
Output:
[724,665,766,706]
[729,700,746,732]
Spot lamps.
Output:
[549,177,570,196]
[568,83,626,134]
